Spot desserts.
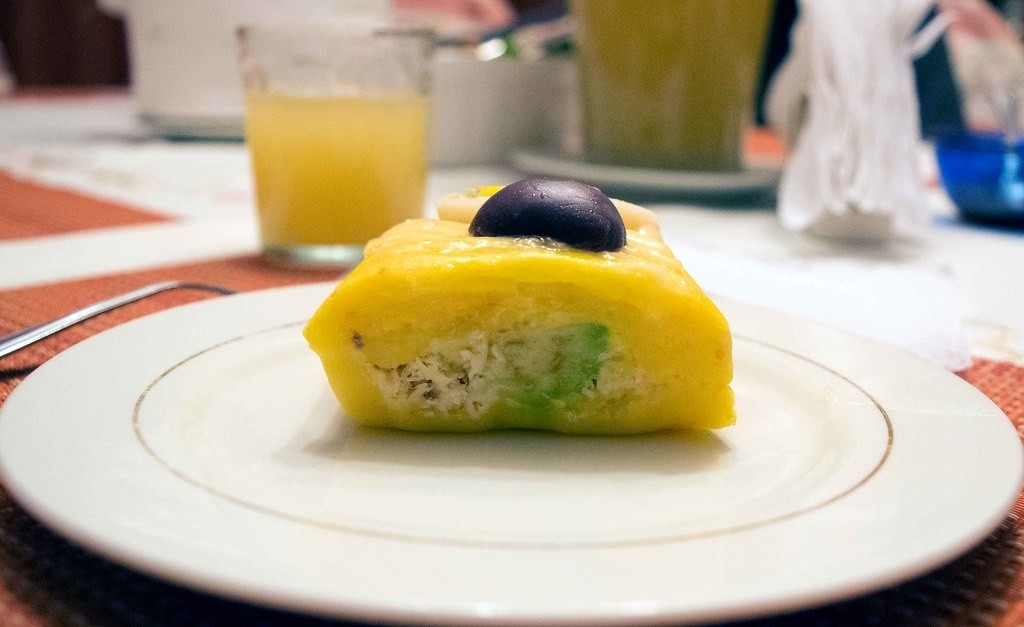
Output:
[302,175,738,433]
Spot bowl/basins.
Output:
[429,64,575,168]
[933,126,1023,229]
[114,1,389,136]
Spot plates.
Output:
[0,283,1024,625]
[516,148,780,200]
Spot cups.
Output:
[568,0,773,167]
[236,26,435,265]
